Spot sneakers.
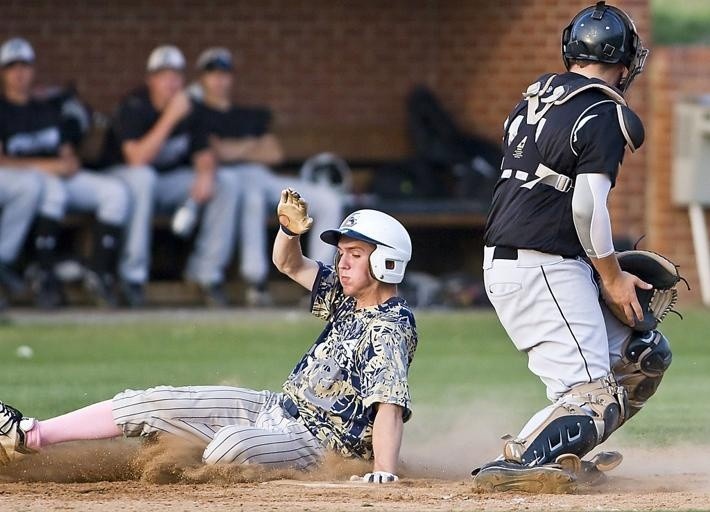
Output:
[471,460,578,494]
[1,401,39,467]
[1,260,274,309]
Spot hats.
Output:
[147,42,187,74]
[0,36,37,67]
[191,46,233,73]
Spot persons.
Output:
[1,37,133,304]
[0,187,419,484]
[100,44,240,310]
[187,49,343,307]
[471,1,674,495]
[0,168,46,304]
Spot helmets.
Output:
[320,208,414,287]
[561,4,649,93]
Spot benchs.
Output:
[0,156,492,282]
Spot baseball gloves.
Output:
[597,250,680,332]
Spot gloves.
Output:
[277,188,314,241]
[348,471,399,484]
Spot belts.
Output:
[491,247,519,261]
